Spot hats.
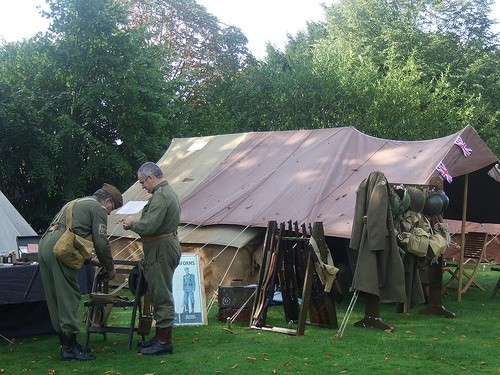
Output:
[184,267,189,270]
[101,183,123,209]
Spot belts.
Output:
[48,225,68,233]
[142,231,178,243]
[185,284,193,286]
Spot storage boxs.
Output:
[218,286,255,310]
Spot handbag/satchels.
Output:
[314,262,339,285]
[53,228,94,270]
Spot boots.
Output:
[59,333,97,360]
[191,303,194,313]
[185,303,189,313]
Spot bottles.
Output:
[0,250,16,266]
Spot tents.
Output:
[106,123,500,338]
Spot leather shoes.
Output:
[138,335,174,355]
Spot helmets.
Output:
[394,186,448,216]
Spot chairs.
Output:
[442,231,488,293]
[84,260,145,351]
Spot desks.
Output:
[0,262,96,337]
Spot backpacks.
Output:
[429,233,448,256]
[407,228,429,258]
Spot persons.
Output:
[36,182,124,360]
[182,267,196,313]
[122,161,182,356]
